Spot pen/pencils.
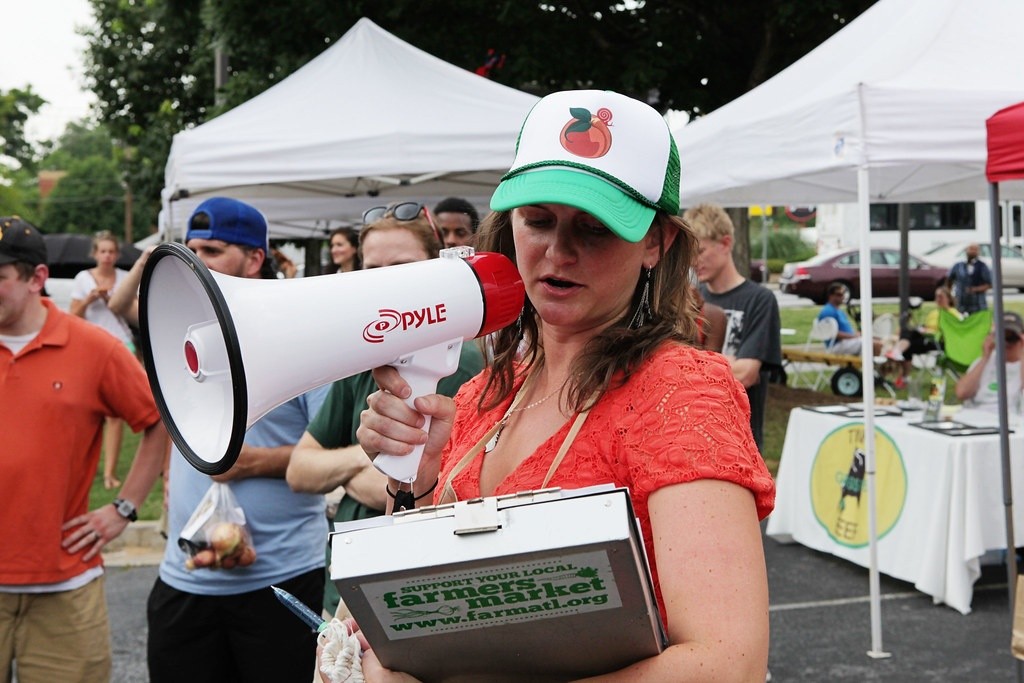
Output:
[270,584,365,658]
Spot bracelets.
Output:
[385,478,439,501]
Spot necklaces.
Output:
[483,370,582,453]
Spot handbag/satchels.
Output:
[179,483,258,570]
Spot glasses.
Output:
[359,201,439,245]
[1003,333,1021,344]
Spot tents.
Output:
[673,1,1023,682]
[157,17,552,239]
[985,102,1023,681]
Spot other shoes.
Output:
[885,349,905,361]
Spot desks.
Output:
[765,399,1024,614]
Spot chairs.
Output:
[783,309,995,401]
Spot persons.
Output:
[67,228,138,491]
[147,195,334,683]
[683,205,780,453]
[0,88,782,683]
[955,311,1024,411]
[317,91,779,683]
[0,215,170,682]
[817,242,993,387]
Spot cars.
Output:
[752,259,770,283]
[779,248,950,303]
[920,242,1024,293]
[41,235,142,278]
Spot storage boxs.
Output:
[327,483,668,683]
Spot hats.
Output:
[999,311,1024,335]
[1,214,48,270]
[488,88,681,242]
[183,196,270,251]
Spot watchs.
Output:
[108,497,139,522]
